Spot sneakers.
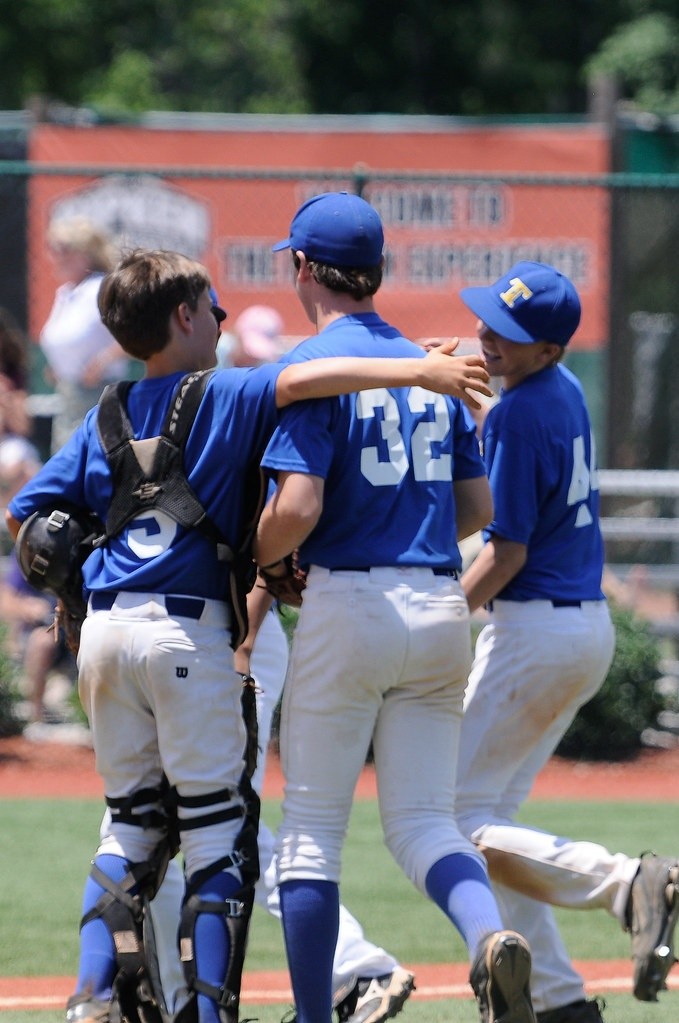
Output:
[625,851,679,1003]
[470,930,537,1023]
[66,991,110,1023]
[535,996,606,1023]
[332,968,416,1023]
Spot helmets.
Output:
[15,510,85,594]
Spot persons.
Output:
[422,262,679,1023]
[247,192,537,1023]
[0,222,493,1023]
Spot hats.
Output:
[272,190,384,267]
[460,261,581,346]
[208,288,227,322]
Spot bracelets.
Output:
[260,561,282,574]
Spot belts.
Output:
[330,566,458,581]
[92,592,206,621]
[484,599,581,612]
